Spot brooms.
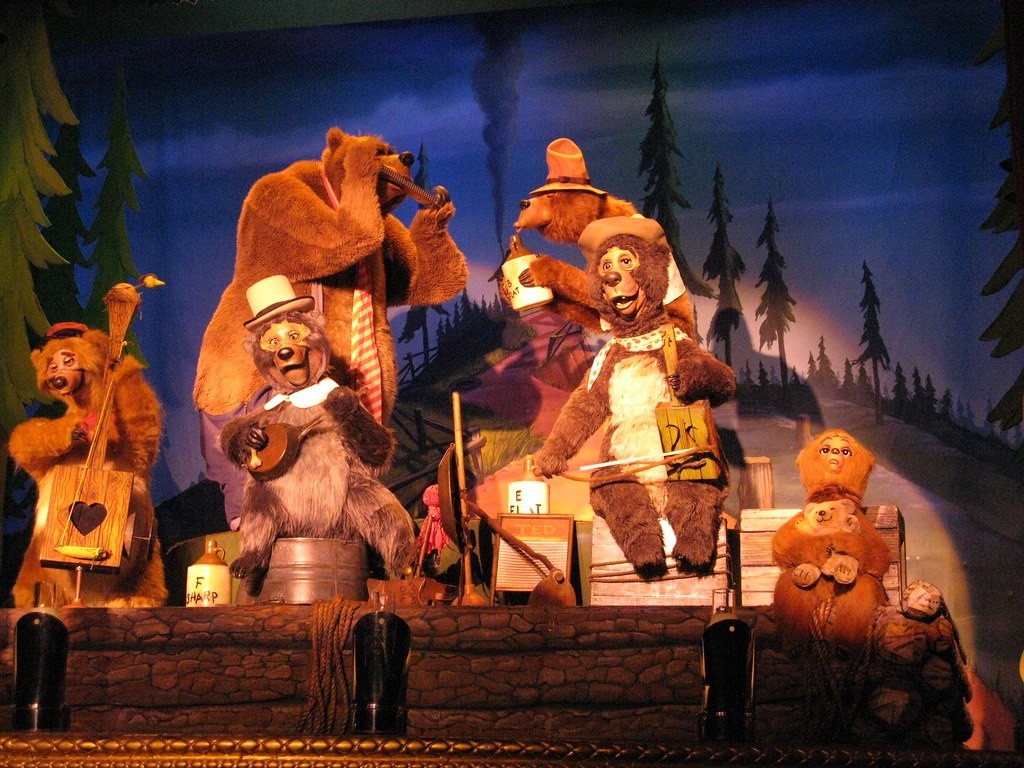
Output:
[464,425,547,607]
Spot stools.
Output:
[740,505,908,610]
[590,513,731,609]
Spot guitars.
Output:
[39,283,141,576]
[247,386,373,479]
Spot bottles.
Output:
[11,582,71,732]
[508,457,550,515]
[186,539,232,607]
[501,234,553,312]
[698,588,756,743]
[350,591,412,734]
